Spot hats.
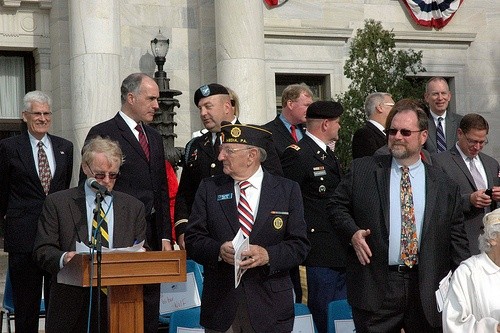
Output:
[306,100,344,119]
[194,83,230,109]
[216,123,273,147]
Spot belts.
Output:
[389,263,418,275]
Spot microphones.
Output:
[86,177,111,196]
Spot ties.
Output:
[90,192,110,249]
[237,180,255,241]
[399,165,418,269]
[436,117,447,153]
[291,124,298,144]
[326,144,335,161]
[37,140,53,195]
[135,123,150,163]
[468,156,490,214]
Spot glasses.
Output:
[218,144,258,155]
[26,111,53,120]
[85,159,122,181]
[462,133,488,150]
[485,213,500,224]
[382,129,423,136]
[230,99,236,107]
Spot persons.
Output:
[442,210,500,333]
[172,84,284,254]
[32,135,145,332]
[256,82,314,307]
[0,90,74,333]
[419,78,466,156]
[182,123,309,333]
[437,112,500,263]
[78,73,175,333]
[190,87,240,137]
[325,97,472,333]
[352,91,394,161]
[286,102,367,333]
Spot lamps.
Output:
[149,29,170,77]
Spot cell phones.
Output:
[485,188,493,199]
